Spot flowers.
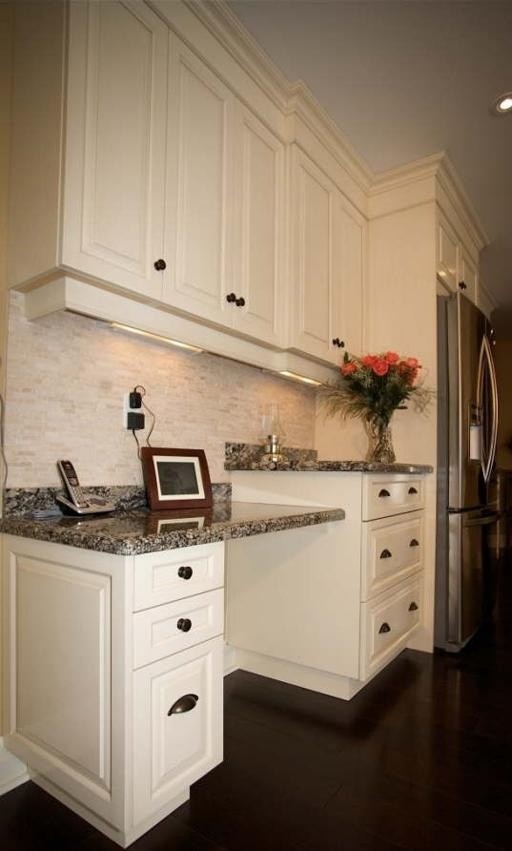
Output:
[318,351,440,420]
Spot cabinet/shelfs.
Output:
[436,225,481,309]
[225,462,435,701]
[0,2,369,386]
[0,530,224,850]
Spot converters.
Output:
[128,412,146,430]
[129,390,142,409]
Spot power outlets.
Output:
[122,390,146,429]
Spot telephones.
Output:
[56,459,116,518]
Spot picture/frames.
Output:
[143,502,213,537]
[141,447,212,512]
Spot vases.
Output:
[364,416,396,462]
